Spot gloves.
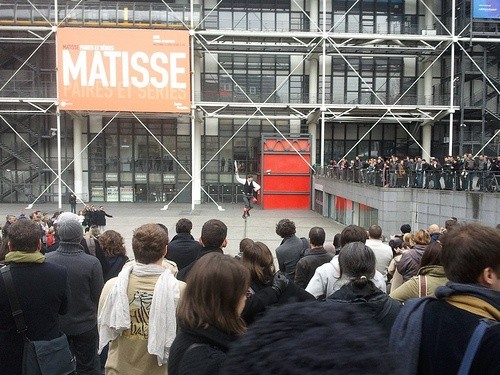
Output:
[271,272,290,298]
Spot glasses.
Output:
[241,289,252,298]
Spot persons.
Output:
[235,160,261,220]
[220,157,225,172]
[45,211,104,375]
[98,230,129,279]
[0,211,102,268]
[388,241,449,302]
[315,152,500,192]
[243,217,500,375]
[163,217,204,271]
[69,192,76,214]
[167,251,250,375]
[228,158,245,173]
[174,219,228,284]
[235,237,254,261]
[275,219,312,284]
[324,242,403,340]
[77,205,112,233]
[98,223,187,375]
[0,219,77,375]
[155,223,168,244]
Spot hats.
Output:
[57,211,85,242]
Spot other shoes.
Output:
[246,211,250,216]
[241,215,246,220]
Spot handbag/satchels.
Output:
[22,331,78,375]
